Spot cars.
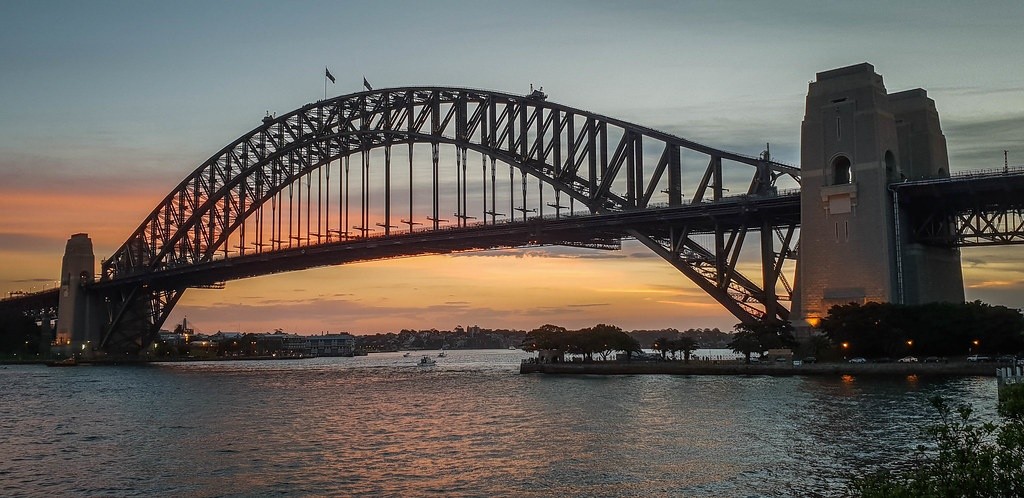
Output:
[922,356,949,364]
[802,357,817,364]
[897,356,918,363]
[995,355,1018,363]
[848,357,867,364]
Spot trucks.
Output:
[966,355,991,363]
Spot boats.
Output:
[402,350,447,367]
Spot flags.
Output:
[326,68,335,83]
[364,78,372,90]
